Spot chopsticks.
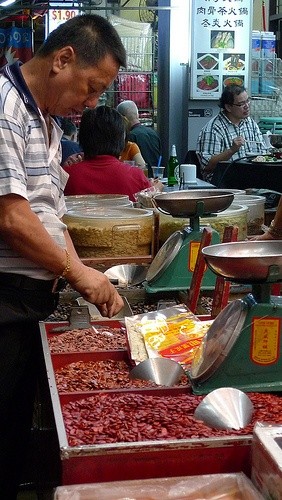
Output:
[245,140,263,144]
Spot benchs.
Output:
[257,116,282,135]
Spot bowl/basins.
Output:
[269,134,282,149]
[63,189,266,258]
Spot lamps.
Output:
[0,0,16,7]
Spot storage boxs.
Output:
[53,471,265,500]
[263,207,277,227]
[251,420,282,500]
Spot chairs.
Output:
[184,149,203,181]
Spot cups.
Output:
[123,161,136,166]
[160,178,168,187]
[180,165,197,185]
[151,167,165,178]
[138,163,148,178]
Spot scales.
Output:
[143,190,231,292]
[189,239,281,395]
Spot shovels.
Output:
[105,264,147,289]
[77,294,134,321]
[128,358,186,388]
[194,387,255,429]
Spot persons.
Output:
[246,195,282,241]
[195,83,273,187]
[54,100,167,203]
[0,13,126,500]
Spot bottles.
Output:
[168,143,179,188]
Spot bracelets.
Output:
[267,220,282,238]
[50,247,71,279]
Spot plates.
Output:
[251,160,282,162]
[223,56,245,70]
[246,153,271,156]
[197,75,219,92]
[197,53,219,70]
[224,77,244,88]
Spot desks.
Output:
[145,176,217,192]
[213,161,282,193]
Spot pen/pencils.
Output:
[153,177,161,182]
